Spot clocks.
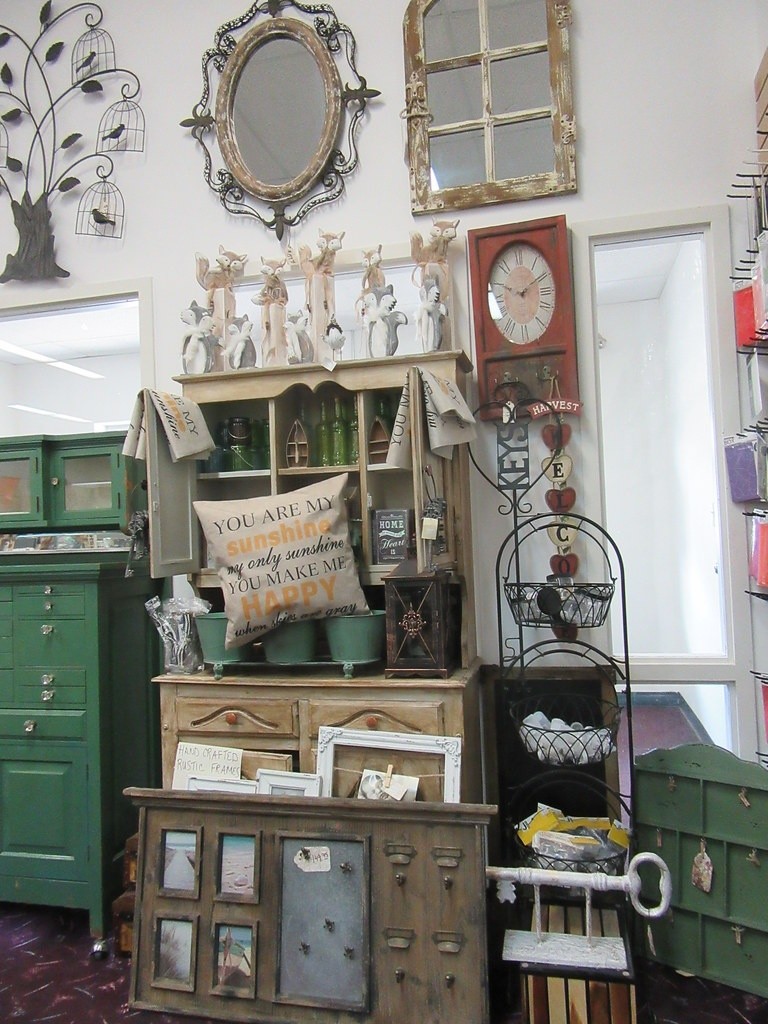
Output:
[467,214,580,420]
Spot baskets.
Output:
[508,649,623,770]
[508,780,628,902]
[501,524,617,628]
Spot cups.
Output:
[520,710,615,766]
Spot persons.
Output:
[361,775,393,800]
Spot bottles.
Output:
[314,400,332,467]
[374,390,391,435]
[348,394,360,465]
[330,396,348,466]
[202,415,271,470]
[297,403,314,466]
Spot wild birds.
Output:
[76,51,96,74]
[101,124,124,141]
[92,209,117,226]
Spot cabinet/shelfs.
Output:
[143,349,484,803]
[1,431,163,963]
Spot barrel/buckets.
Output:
[264,616,318,663]
[194,612,253,663]
[323,609,386,663]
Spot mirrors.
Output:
[180,0,381,242]
